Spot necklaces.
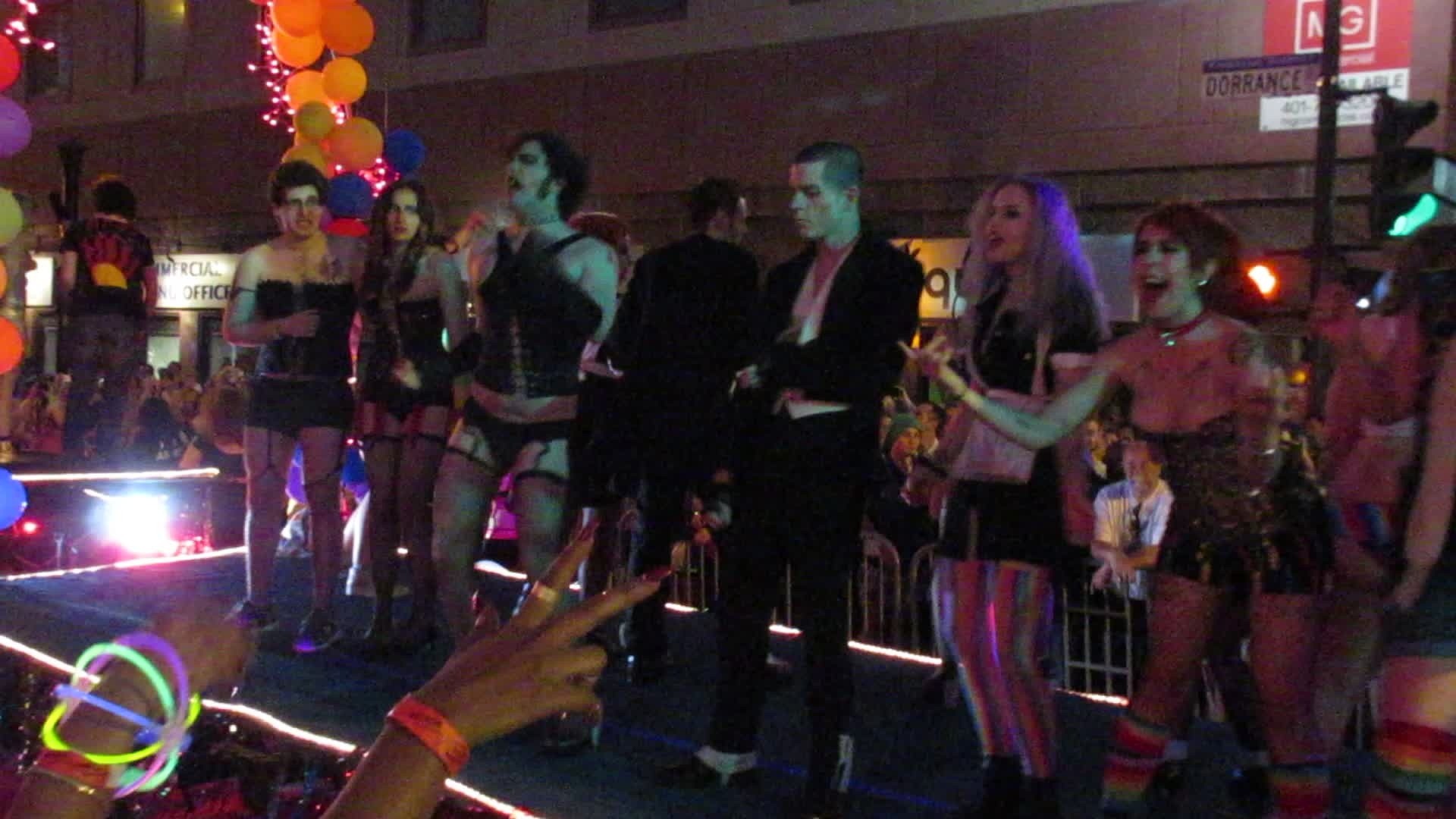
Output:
[1146,308,1210,349]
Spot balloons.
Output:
[0,1,37,533]
[265,0,425,265]
[271,419,524,543]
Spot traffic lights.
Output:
[1376,94,1444,239]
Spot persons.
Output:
[1322,226,1456,817]
[0,589,252,819]
[218,163,369,659]
[895,201,1325,819]
[589,175,765,687]
[565,210,632,405]
[305,521,677,818]
[431,131,621,715]
[900,173,1111,816]
[644,354,1347,708]
[353,180,473,666]
[662,142,932,795]
[0,175,253,476]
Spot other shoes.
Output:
[219,566,1058,819]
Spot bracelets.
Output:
[382,691,469,777]
[22,624,202,808]
[270,319,284,342]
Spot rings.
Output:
[527,581,562,607]
